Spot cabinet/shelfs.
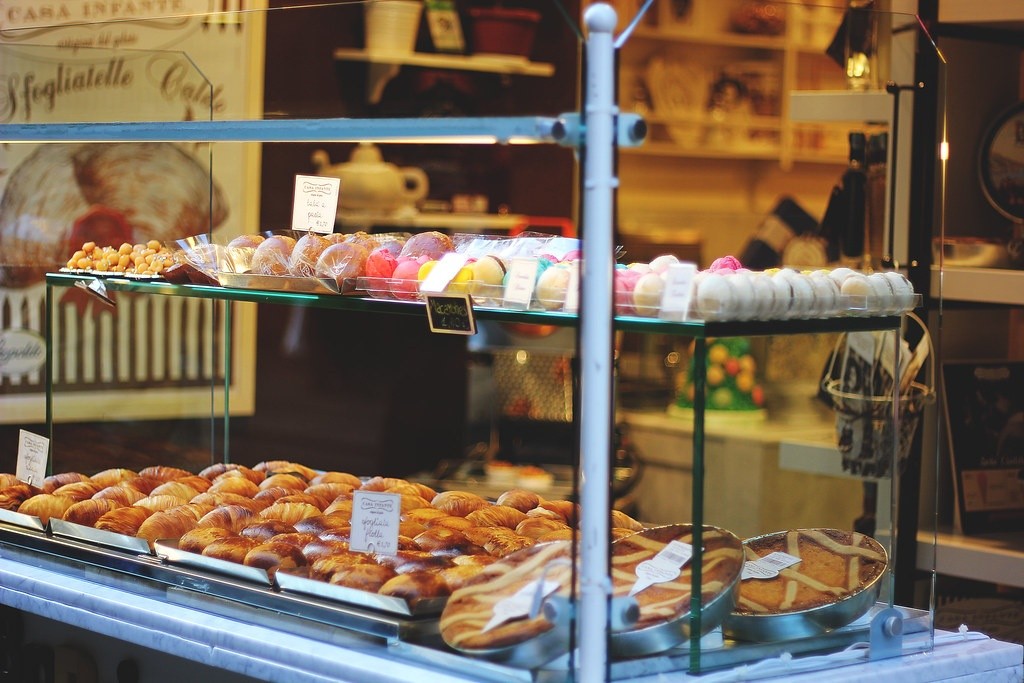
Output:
[573,0,902,178]
[616,426,871,546]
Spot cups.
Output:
[364,0,424,55]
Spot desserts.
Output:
[221,231,915,323]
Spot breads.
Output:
[0,461,645,605]
[440,522,886,651]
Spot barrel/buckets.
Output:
[827,380,930,481]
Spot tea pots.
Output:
[311,142,428,216]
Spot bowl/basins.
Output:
[467,8,542,60]
